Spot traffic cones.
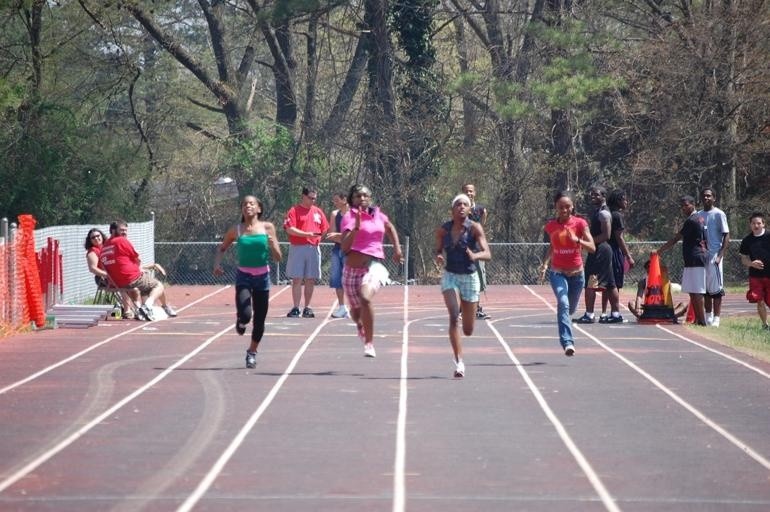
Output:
[686,298,696,322]
[639,248,681,326]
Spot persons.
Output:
[572,186,730,355]
[435,194,491,377]
[283,186,330,318]
[457,183,491,322]
[213,194,282,368]
[325,190,350,319]
[340,183,405,357]
[537,190,596,356]
[738,212,770,331]
[84,221,178,321]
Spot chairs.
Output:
[92,265,151,320]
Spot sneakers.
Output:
[246,350,256,368]
[476,311,490,319]
[140,304,176,320]
[287,306,348,317]
[364,343,376,357]
[237,319,245,334]
[454,364,464,376]
[565,345,574,355]
[573,313,623,323]
